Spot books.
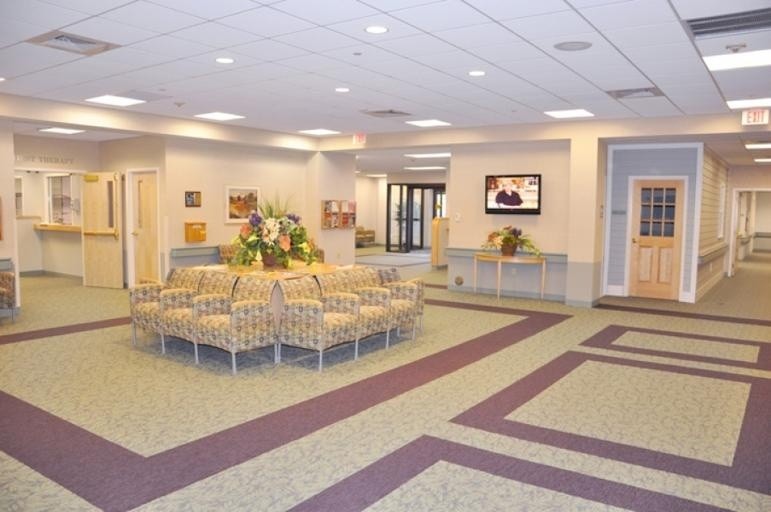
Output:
[325,201,357,228]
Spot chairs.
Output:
[130,265,425,376]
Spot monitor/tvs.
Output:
[483,173,541,216]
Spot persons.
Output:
[496,180,525,208]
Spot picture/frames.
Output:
[486,174,541,215]
[185,191,201,207]
[226,187,261,225]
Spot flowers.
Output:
[480,225,542,258]
[227,189,322,265]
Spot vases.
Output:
[500,244,516,257]
[260,249,288,270]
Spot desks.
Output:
[473,252,547,302]
